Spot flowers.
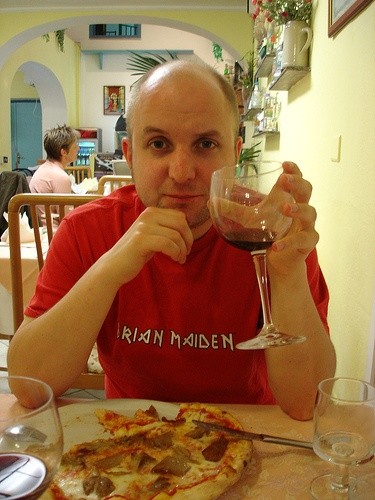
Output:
[250,0,317,23]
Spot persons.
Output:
[6,59,337,422]
[29,122,81,228]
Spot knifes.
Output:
[191,419,314,449]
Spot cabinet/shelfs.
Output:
[240,107,280,138]
[255,52,310,91]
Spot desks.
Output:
[0,227,60,336]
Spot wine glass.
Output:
[309,376,375,500]
[209,160,307,351]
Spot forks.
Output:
[4,424,48,443]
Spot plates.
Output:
[0,398,181,500]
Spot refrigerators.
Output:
[72,129,102,173]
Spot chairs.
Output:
[4,189,114,338]
[57,163,93,187]
[96,172,137,194]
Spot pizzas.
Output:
[36,403,253,500]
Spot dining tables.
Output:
[0,378,375,500]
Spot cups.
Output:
[0,375,64,500]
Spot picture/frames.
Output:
[326,0,375,39]
[102,84,127,118]
